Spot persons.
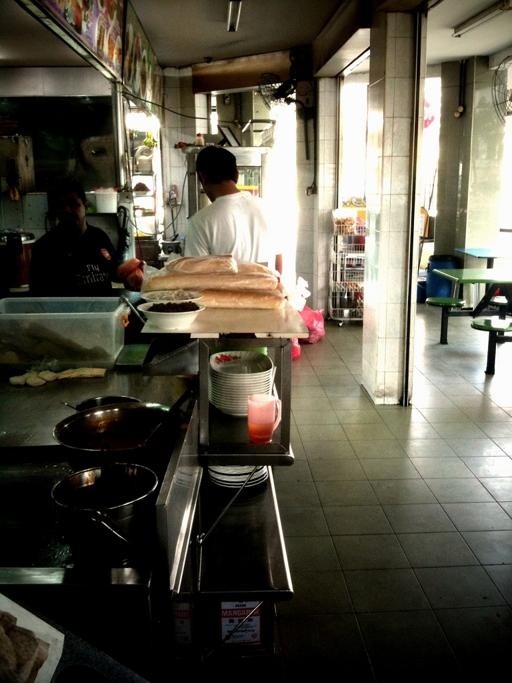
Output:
[181,144,285,296]
[28,180,128,296]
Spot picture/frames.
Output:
[217,124,240,147]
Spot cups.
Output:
[247,394,282,444]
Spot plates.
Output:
[208,465,270,489]
[208,350,274,417]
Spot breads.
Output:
[143,254,287,310]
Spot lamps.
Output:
[122,91,159,133]
[226,0,243,32]
[452,1,512,39]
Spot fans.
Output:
[256,69,314,120]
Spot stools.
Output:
[427,297,464,345]
[470,315,512,375]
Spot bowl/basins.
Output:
[141,290,204,303]
[137,302,206,329]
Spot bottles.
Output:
[195,133,204,145]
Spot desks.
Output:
[444,248,510,292]
[435,267,512,318]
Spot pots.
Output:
[49,462,160,533]
[52,387,195,460]
